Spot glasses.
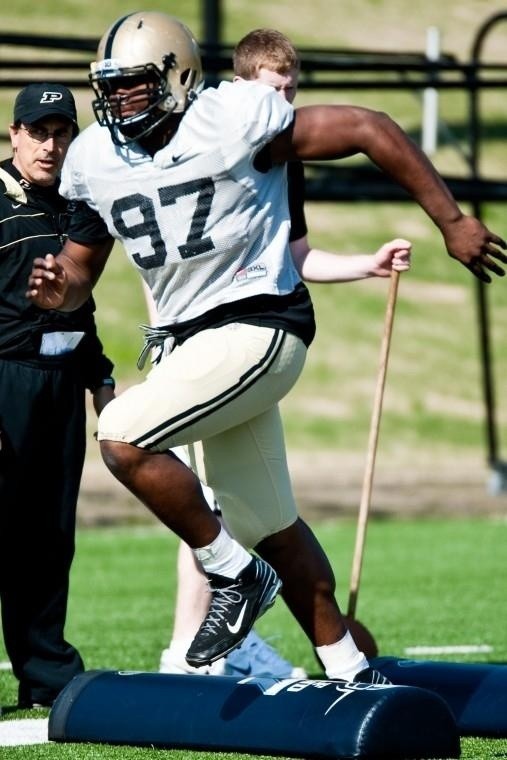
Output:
[19,126,70,145]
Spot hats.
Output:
[14,83,79,133]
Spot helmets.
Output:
[88,12,205,146]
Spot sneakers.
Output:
[185,552,283,668]
[331,665,392,685]
[159,633,291,676]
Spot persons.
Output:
[0,80,120,704]
[25,12,507,685]
[154,28,415,679]
